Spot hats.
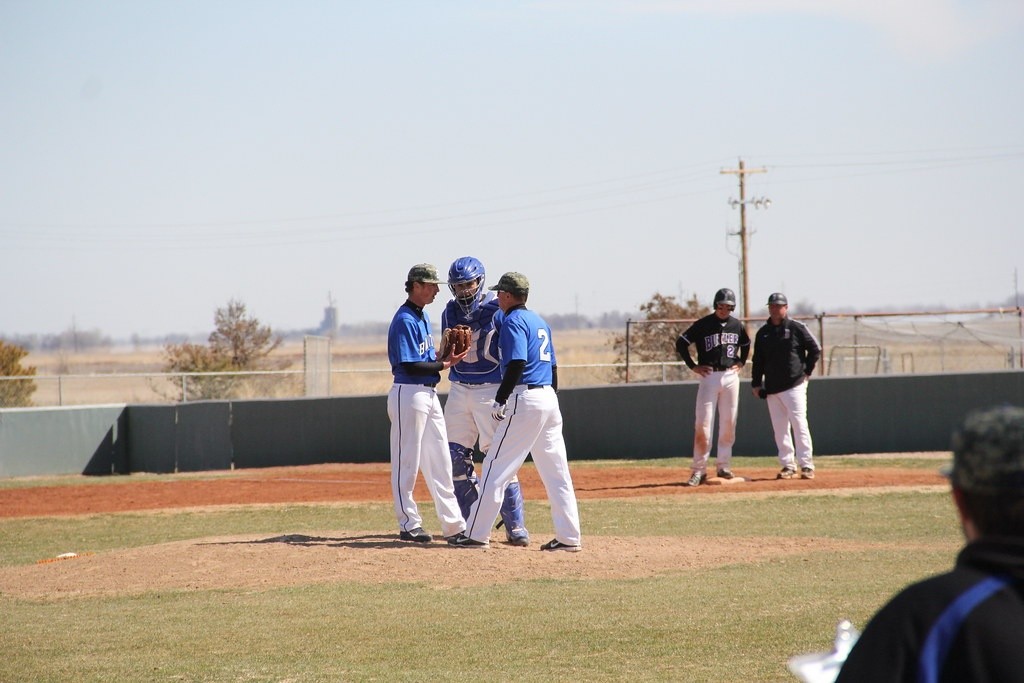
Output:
[939,408,1024,497]
[487,271,529,295]
[408,263,448,284]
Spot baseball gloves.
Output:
[438,323,473,360]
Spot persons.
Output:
[441,256,529,543]
[832,407,1024,683]
[448,269,582,551]
[675,288,751,486]
[383,263,471,546]
[749,292,821,479]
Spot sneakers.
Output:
[717,468,735,478]
[447,530,466,538]
[687,472,705,488]
[778,466,798,480]
[801,466,814,480]
[506,529,528,545]
[540,537,582,552]
[448,536,488,549]
[400,527,432,542]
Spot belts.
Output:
[712,366,727,372]
[459,380,484,386]
[528,383,543,389]
[424,383,436,388]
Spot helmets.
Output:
[448,257,485,314]
[713,288,736,312]
[767,292,788,305]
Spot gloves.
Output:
[490,402,506,422]
